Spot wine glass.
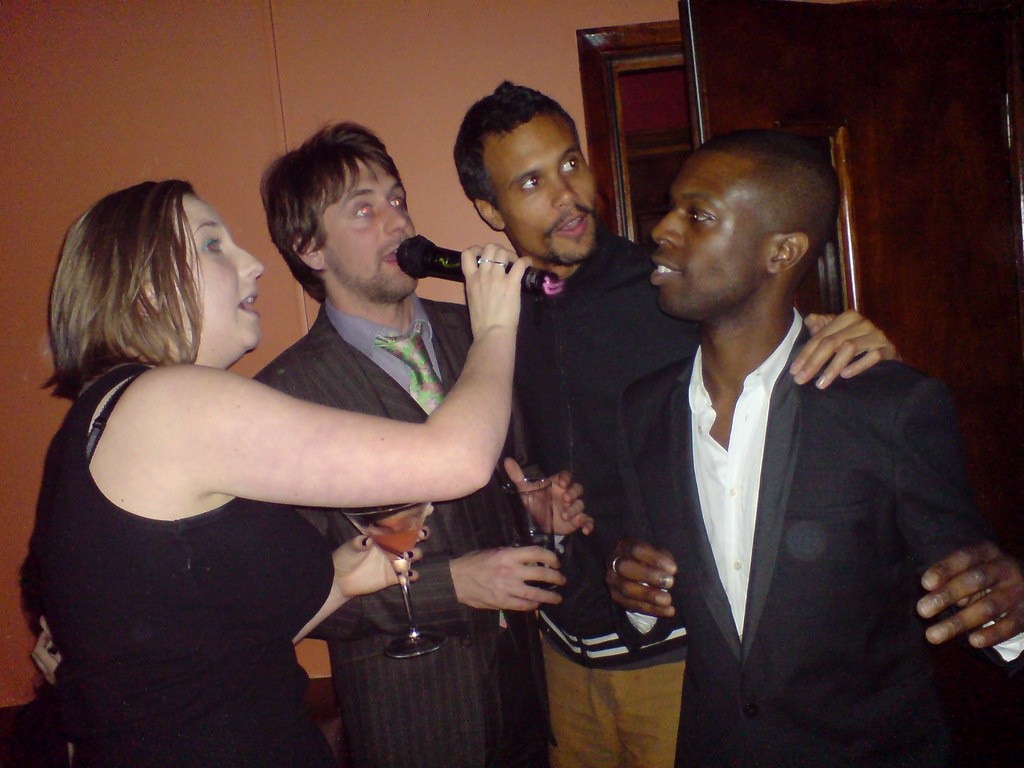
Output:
[339,500,449,659]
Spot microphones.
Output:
[397,234,560,296]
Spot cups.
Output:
[495,475,558,591]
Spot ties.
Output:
[371,321,447,417]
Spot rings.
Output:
[478,238,493,264]
[494,262,507,269]
[612,555,622,574]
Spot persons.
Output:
[453,80,1024,768]
[19,121,594,768]
